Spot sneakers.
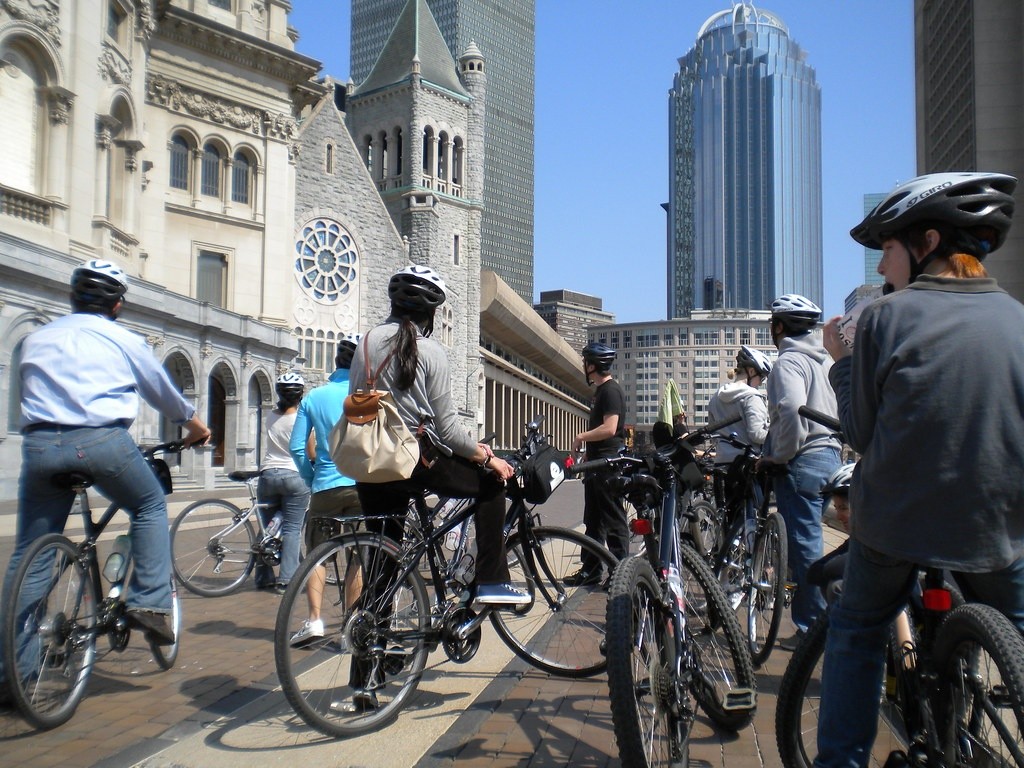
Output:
[563,565,611,591]
[124,607,176,645]
[475,583,531,603]
[352,687,378,712]
[289,619,324,647]
[0,684,16,715]
[340,636,348,649]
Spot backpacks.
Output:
[328,328,429,483]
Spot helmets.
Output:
[770,294,822,329]
[736,345,773,373]
[819,462,857,494]
[388,265,446,307]
[338,332,362,368]
[276,373,304,395]
[582,343,616,365]
[71,259,128,300]
[850,172,1018,253]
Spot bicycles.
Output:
[776,405,1024,768]
[273,415,635,739]
[622,449,985,741]
[565,413,758,767]
[168,477,535,617]
[4,435,206,729]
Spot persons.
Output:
[289,333,364,653]
[564,452,584,479]
[814,174,1024,768]
[572,342,628,590]
[256,370,313,594]
[349,265,533,708]
[761,294,844,651]
[708,346,774,594]
[0,259,212,711]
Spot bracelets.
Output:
[478,445,491,467]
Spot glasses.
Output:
[753,366,765,382]
[768,318,773,324]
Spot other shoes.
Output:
[276,584,288,594]
[780,629,801,650]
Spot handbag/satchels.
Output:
[525,444,567,504]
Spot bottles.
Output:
[455,524,510,586]
[444,523,461,551]
[744,508,756,553]
[432,498,457,527]
[265,510,284,537]
[664,562,686,629]
[102,535,132,582]
